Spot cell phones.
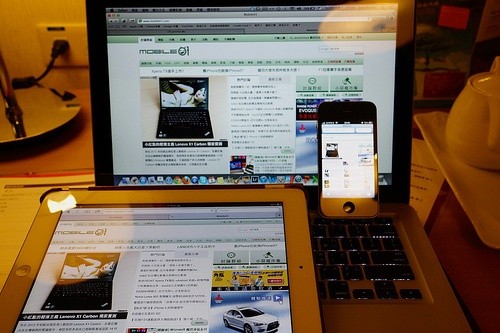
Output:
[316,101,379,219]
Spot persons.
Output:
[230,275,240,286]
[60,257,117,280]
[161,82,207,107]
[254,275,263,286]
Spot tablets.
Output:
[0,188,323,332]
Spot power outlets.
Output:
[36,23,89,68]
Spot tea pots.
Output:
[442,57,500,173]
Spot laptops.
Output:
[85,0,472,333]
